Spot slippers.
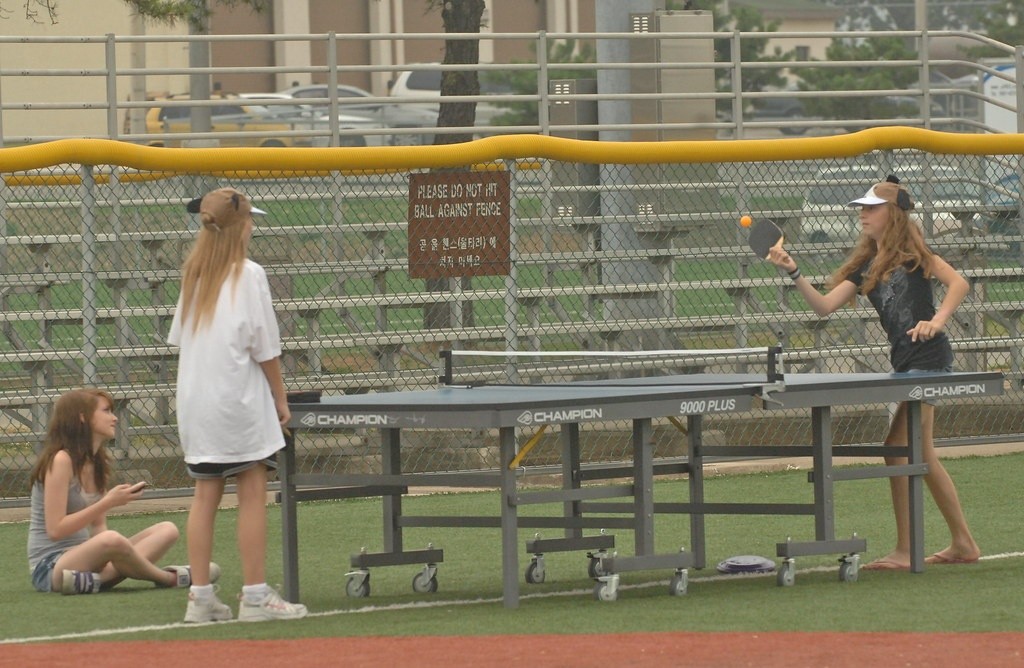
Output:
[923,553,978,563]
[862,557,915,570]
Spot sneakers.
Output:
[183,592,233,622]
[155,562,221,591]
[63,569,101,595]
[237,592,309,622]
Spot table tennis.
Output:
[740,215,752,227]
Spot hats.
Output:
[199,187,268,232]
[846,174,915,210]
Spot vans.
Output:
[389,62,526,143]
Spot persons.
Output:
[165,188,311,624]
[763,175,979,572]
[26,387,222,596]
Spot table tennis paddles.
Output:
[748,217,785,259]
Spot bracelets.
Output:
[787,266,800,280]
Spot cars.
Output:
[146,83,441,147]
